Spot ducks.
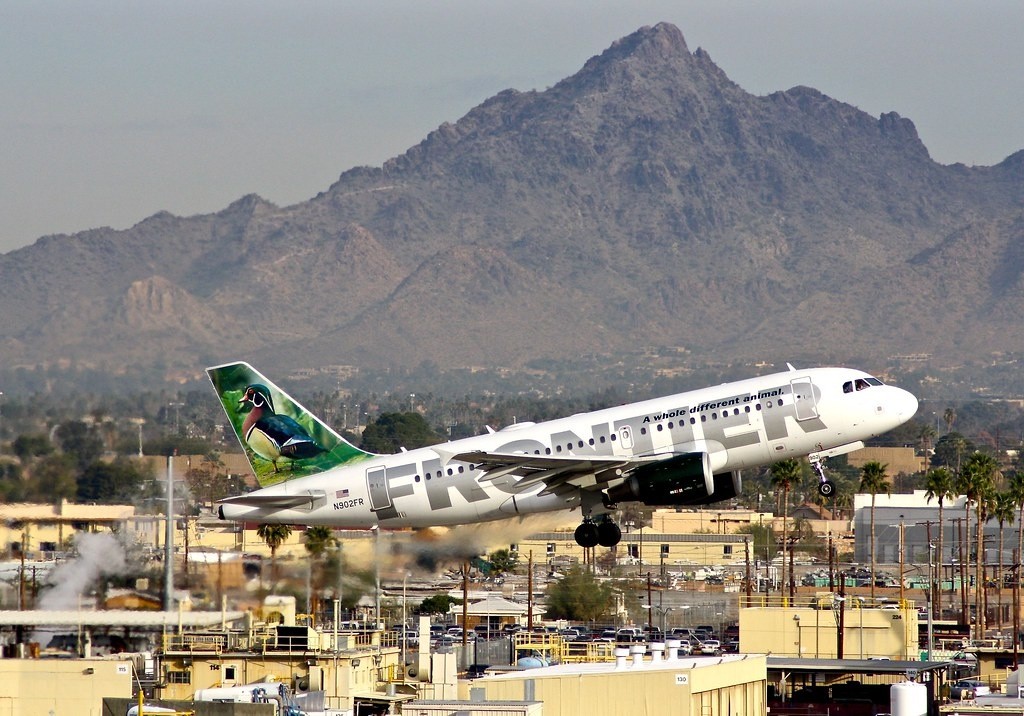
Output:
[238,384,330,476]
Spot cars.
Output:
[467,665,491,679]
[431,625,447,635]
[393,625,408,632]
[474,625,493,637]
[399,631,419,646]
[436,637,454,647]
[502,623,592,643]
[431,635,445,646]
[724,626,739,652]
[950,680,991,699]
[598,627,675,643]
[678,640,694,655]
[448,628,463,637]
[691,629,708,640]
[701,640,721,654]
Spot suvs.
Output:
[672,628,690,640]
[456,630,477,643]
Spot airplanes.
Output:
[205,361,920,549]
